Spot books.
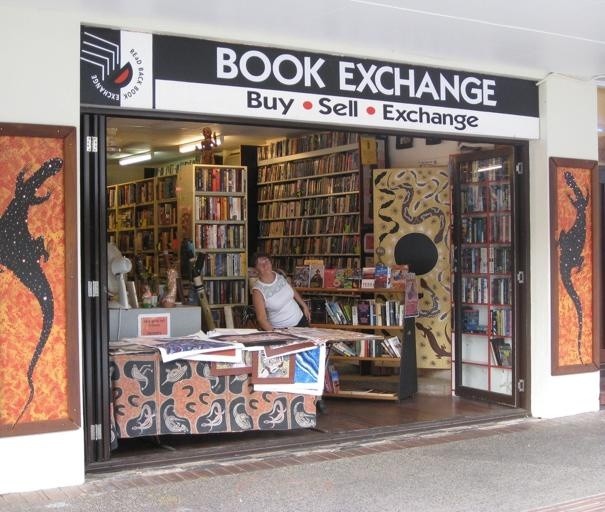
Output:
[194,167,246,306]
[256,131,362,220]
[106,176,177,298]
[292,257,405,359]
[256,216,360,278]
[455,158,512,367]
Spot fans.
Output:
[107,243,133,310]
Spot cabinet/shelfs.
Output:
[106,174,176,284]
[177,163,249,333]
[451,156,512,397]
[144,156,222,178]
[285,278,418,403]
[240,134,389,275]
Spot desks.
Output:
[109,328,384,451]
[108,306,202,341]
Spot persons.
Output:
[252,253,312,331]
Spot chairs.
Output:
[249,278,259,290]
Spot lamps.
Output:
[118,152,154,166]
[179,135,225,154]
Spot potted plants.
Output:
[134,249,160,308]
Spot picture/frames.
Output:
[308,264,324,288]
[1,122,81,436]
[549,157,599,375]
